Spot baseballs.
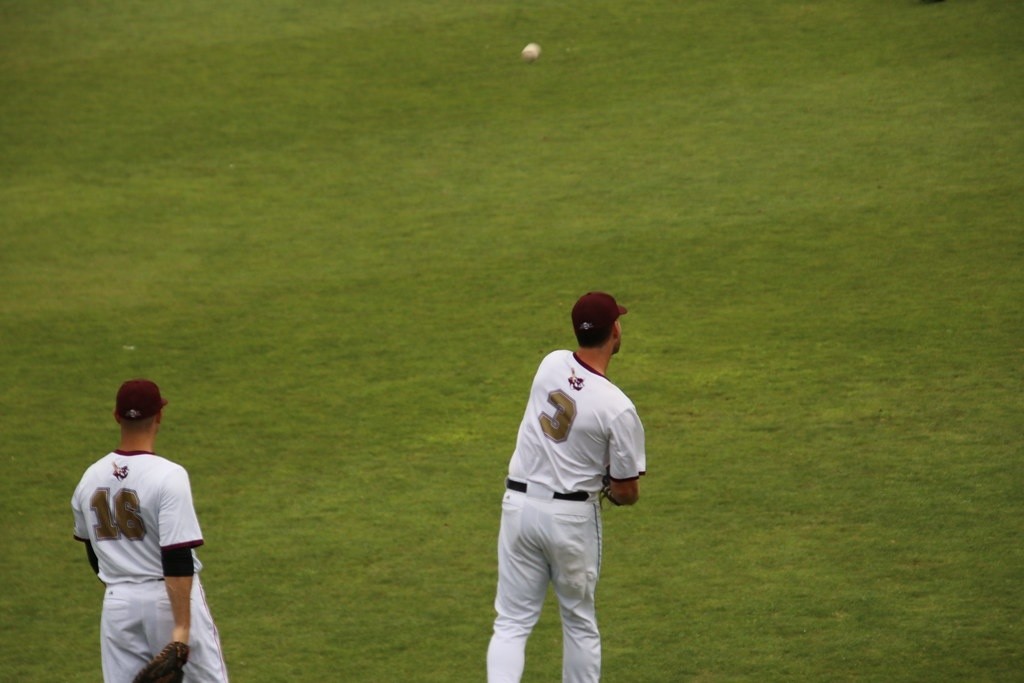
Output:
[522,43,540,62]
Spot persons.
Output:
[486,292,647,683]
[70,379,228,683]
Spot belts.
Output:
[507,480,589,502]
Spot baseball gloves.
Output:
[131,642,189,683]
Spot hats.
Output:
[116,380,168,420]
[571,292,627,335]
